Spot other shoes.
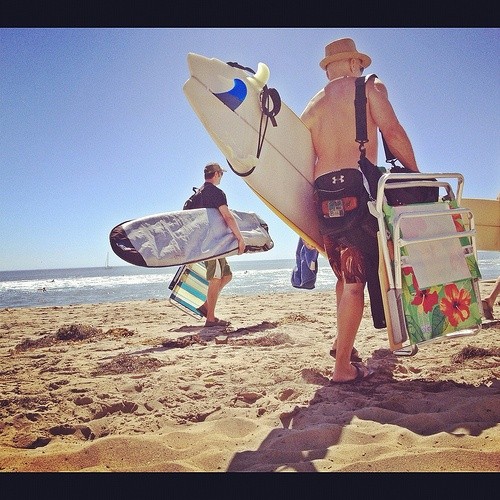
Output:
[196,305,219,321]
[206,317,231,327]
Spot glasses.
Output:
[356,62,364,75]
[218,171,224,176]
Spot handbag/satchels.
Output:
[359,156,439,207]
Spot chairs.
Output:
[168,261,211,320]
[367,171,485,357]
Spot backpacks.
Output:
[182,185,217,210]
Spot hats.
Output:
[205,163,228,175]
[320,38,371,71]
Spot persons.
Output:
[481,193,500,320]
[197,162,245,326]
[300,39,421,382]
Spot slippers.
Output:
[481,299,494,320]
[330,346,362,362]
[331,363,375,383]
